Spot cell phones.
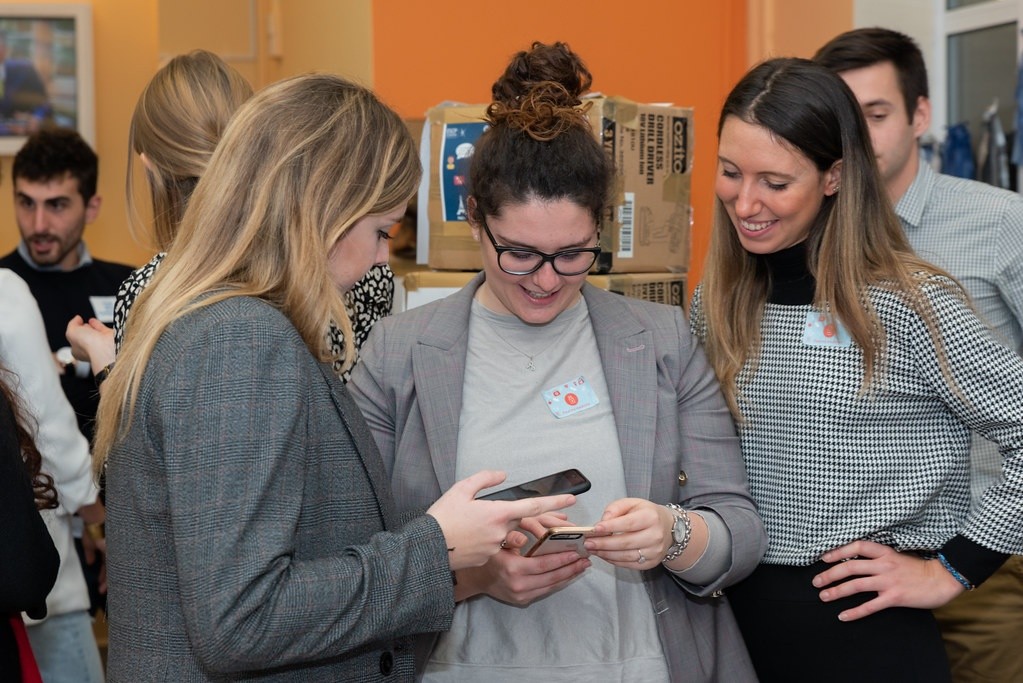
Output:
[525,528,612,559]
[474,468,590,501]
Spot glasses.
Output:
[475,204,603,276]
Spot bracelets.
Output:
[664,498,693,562]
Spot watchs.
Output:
[661,503,688,561]
[94,360,116,394]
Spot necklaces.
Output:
[475,279,586,374]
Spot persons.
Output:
[688,55,1022,682]
[0,352,65,682]
[0,124,146,650]
[91,75,580,682]
[806,25,1022,682]
[0,261,110,682]
[60,48,401,401]
[338,33,774,683]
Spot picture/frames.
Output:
[0,3,96,157]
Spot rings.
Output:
[500,539,509,551]
[634,547,647,566]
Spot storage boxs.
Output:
[420,94,689,271]
